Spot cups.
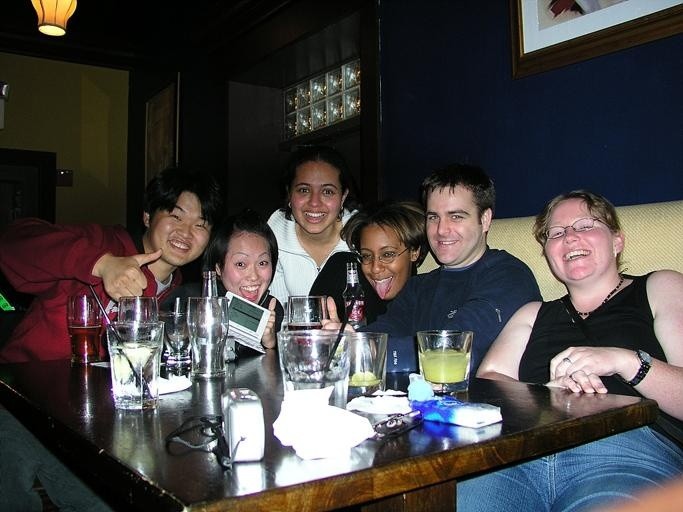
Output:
[104,320,163,410]
[415,326,471,393]
[186,296,230,378]
[346,332,390,400]
[190,379,223,434]
[273,328,351,411]
[110,411,161,484]
[156,311,197,364]
[115,295,158,323]
[67,367,102,415]
[286,293,328,329]
[66,293,102,367]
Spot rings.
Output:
[562,357,570,363]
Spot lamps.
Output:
[30,0,79,38]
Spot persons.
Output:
[308,201,429,326]
[321,164,542,380]
[455,189,682,510]
[0,164,223,510]
[162,213,285,361]
[267,140,359,308]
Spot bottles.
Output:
[342,260,366,327]
[293,68,360,135]
[199,269,219,297]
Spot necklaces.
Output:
[576,268,627,314]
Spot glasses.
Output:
[356,248,410,266]
[545,216,610,239]
[372,408,423,435]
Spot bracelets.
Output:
[260,337,276,350]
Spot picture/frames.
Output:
[141,72,185,190]
[506,1,681,80]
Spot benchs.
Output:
[403,199,682,305]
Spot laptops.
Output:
[223,289,271,355]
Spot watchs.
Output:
[623,349,653,385]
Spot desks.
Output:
[0,358,659,512]
